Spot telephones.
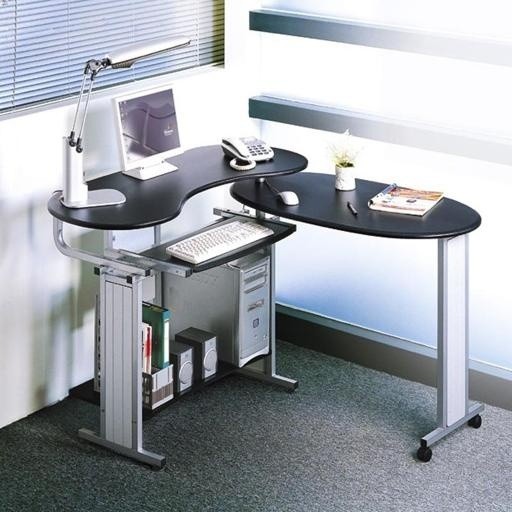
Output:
[221,134,274,162]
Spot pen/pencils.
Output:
[347,202,358,215]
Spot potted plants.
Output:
[332,131,360,191]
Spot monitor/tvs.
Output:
[114,84,184,181]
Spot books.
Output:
[142,300,170,370]
[367,183,445,216]
[141,321,153,374]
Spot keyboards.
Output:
[165,219,275,264]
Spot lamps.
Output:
[59,35,192,210]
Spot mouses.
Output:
[276,191,300,206]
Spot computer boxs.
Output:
[162,252,270,368]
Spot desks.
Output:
[227,168,488,464]
[48,142,310,466]
